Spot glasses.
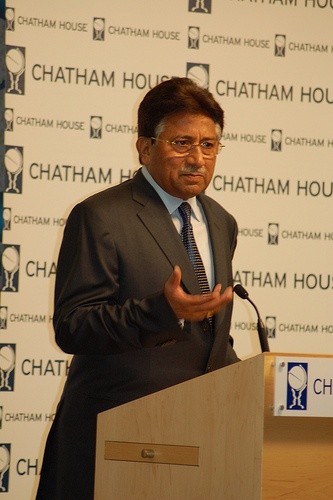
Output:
[150,137,225,154]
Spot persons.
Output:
[35,76,242,500]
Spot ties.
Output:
[178,203,214,329]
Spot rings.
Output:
[207,311,213,319]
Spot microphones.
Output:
[233,285,270,352]
